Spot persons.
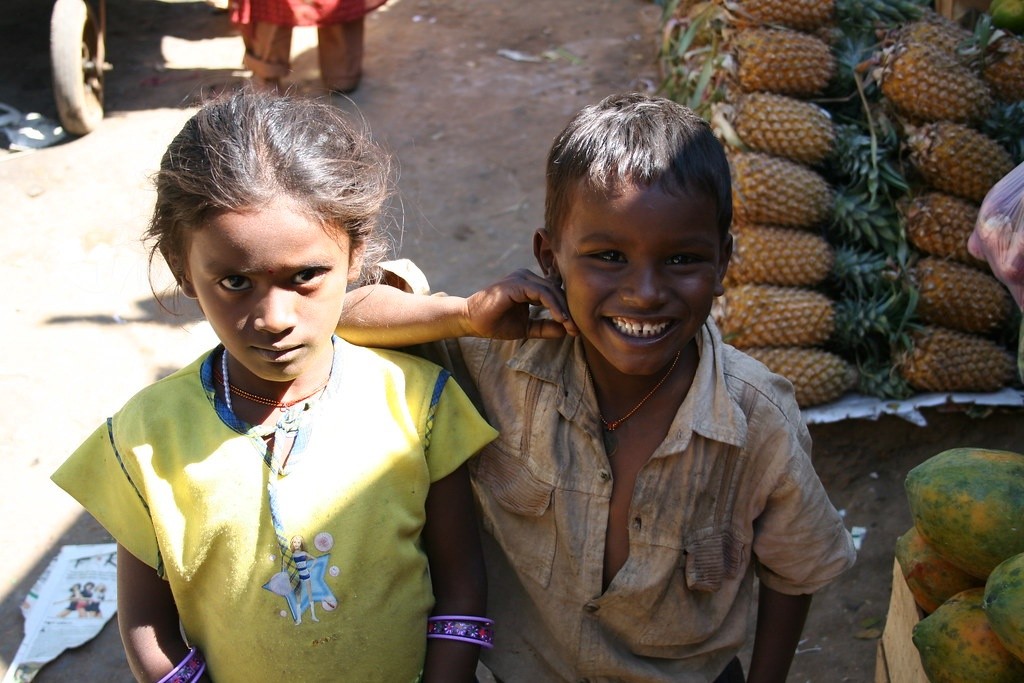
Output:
[210,0,388,96]
[336,92,856,683]
[51,90,502,683]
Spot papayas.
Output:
[895,446,1024,683]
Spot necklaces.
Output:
[223,350,233,414]
[600,352,681,457]
[213,369,328,412]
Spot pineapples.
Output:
[674,0,1024,405]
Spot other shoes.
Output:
[209,78,281,102]
[332,64,362,94]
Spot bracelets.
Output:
[427,615,495,648]
[159,646,204,683]
